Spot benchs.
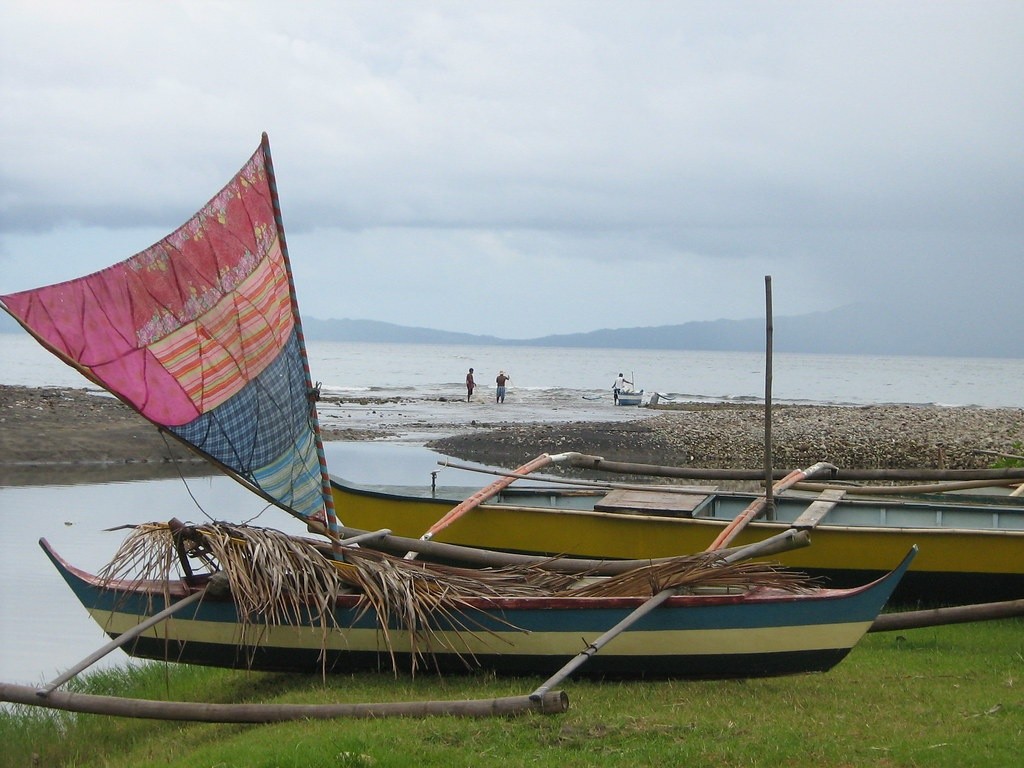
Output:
[792,488,846,529]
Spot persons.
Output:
[466,368,475,402]
[612,373,633,406]
[496,371,509,403]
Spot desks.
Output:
[594,484,719,516]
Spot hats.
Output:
[500,371,504,374]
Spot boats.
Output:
[617,372,644,405]
[0,129,916,681]
[328,275,1023,606]
